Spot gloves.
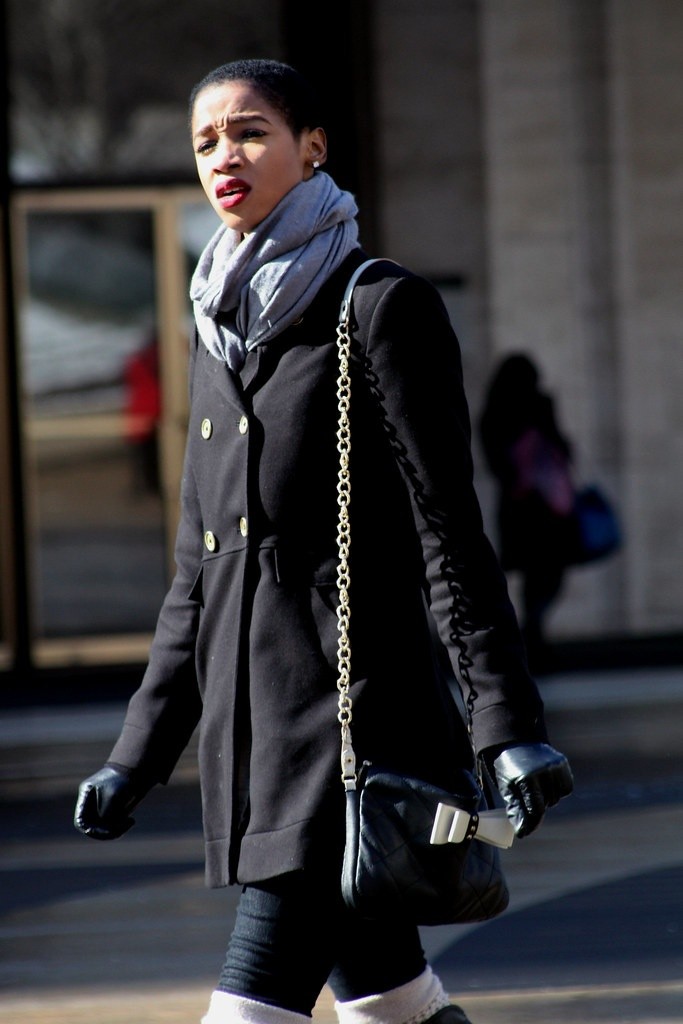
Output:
[73,769,140,842]
[490,744,572,837]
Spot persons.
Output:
[476,350,580,668]
[73,63,571,1024]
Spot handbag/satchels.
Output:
[568,481,627,569]
[342,759,509,926]
